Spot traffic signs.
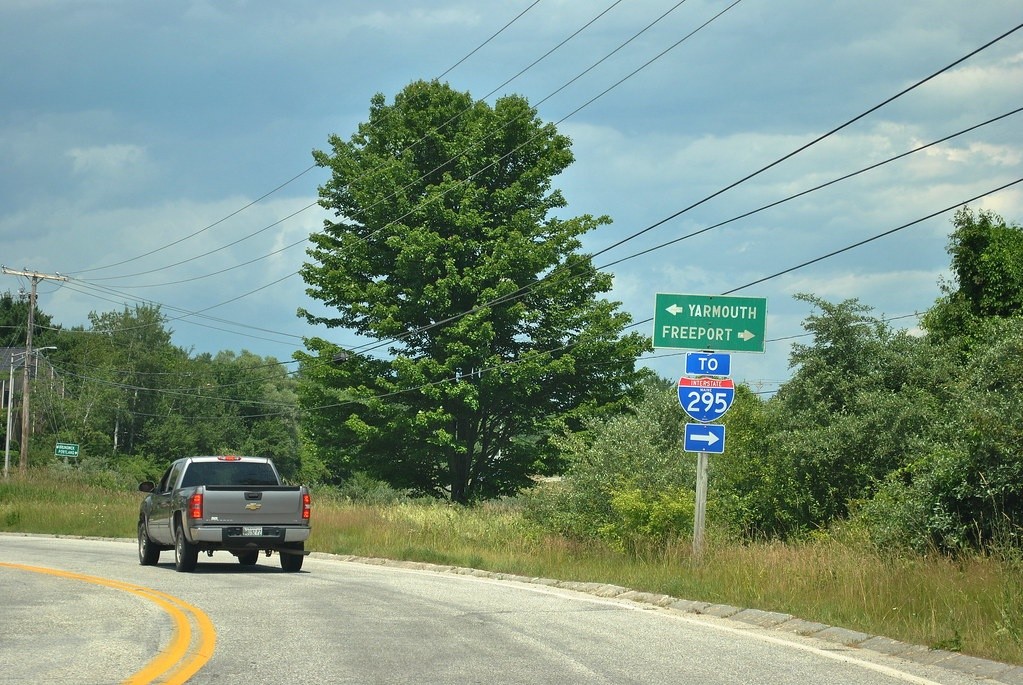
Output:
[652,292,768,353]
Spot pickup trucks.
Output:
[137,455,312,574]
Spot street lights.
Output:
[3,346,58,480]
